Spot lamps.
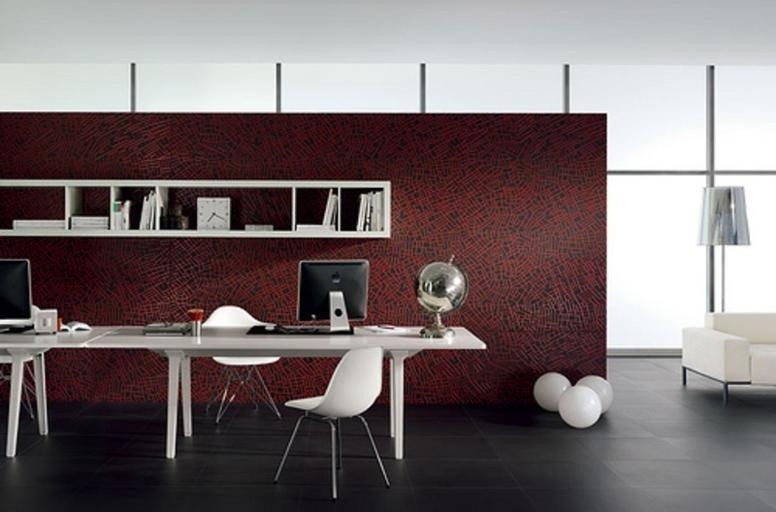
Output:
[696,186,750,314]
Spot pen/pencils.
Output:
[378,325,395,329]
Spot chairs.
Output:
[274,346,390,499]
[0,305,50,461]
[201,305,280,424]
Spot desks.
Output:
[0,327,486,460]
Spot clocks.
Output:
[196,197,230,231]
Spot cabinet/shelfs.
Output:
[0,179,391,237]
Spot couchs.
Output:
[682,313,775,403]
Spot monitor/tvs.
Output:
[0,258,33,328]
[296,259,370,334]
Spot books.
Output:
[141,321,191,331]
[69,186,170,231]
[59,319,91,334]
[12,219,64,229]
[142,328,191,336]
[296,186,385,232]
[244,223,274,231]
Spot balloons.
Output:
[532,370,573,413]
[558,385,603,431]
[573,373,614,416]
